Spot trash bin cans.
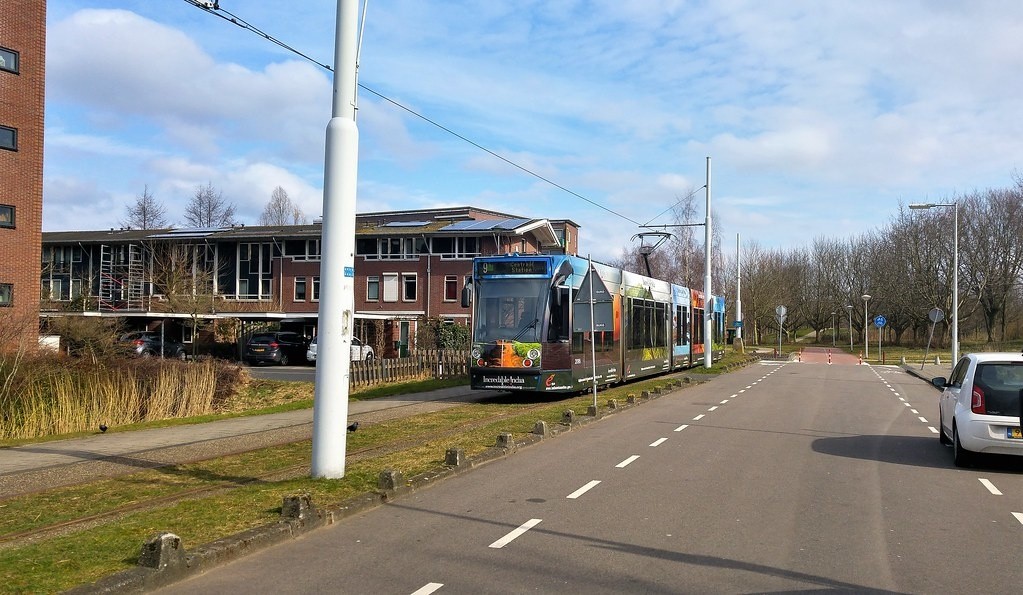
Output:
[400,345,408,357]
[395,341,400,349]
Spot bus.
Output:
[460,231,726,397]
[726,327,744,344]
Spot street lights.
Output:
[861,295,872,359]
[847,305,854,351]
[909,202,960,369]
[831,311,838,347]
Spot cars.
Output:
[113,330,189,362]
[307,335,374,365]
[931,352,1023,469]
[245,331,309,366]
